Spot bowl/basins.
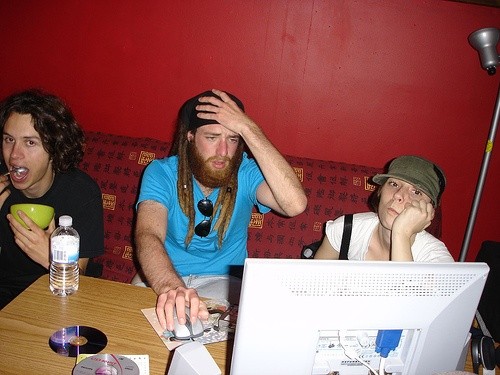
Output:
[10,204,55,230]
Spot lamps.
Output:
[458,27,500,262]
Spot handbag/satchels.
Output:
[301,214,353,260]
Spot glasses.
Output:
[195,197,213,238]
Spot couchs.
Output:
[73,130,443,284]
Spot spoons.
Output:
[4,167,25,176]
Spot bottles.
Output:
[304,249,314,259]
[49,215,79,296]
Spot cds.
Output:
[72,353,139,375]
[49,326,107,357]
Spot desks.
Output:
[0,273,500,375]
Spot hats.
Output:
[372,155,446,209]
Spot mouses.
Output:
[172,306,204,339]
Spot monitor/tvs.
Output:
[229,257,489,375]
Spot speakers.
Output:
[168,341,221,375]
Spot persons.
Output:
[313,155,455,263]
[0,86,107,307]
[126,87,308,331]
